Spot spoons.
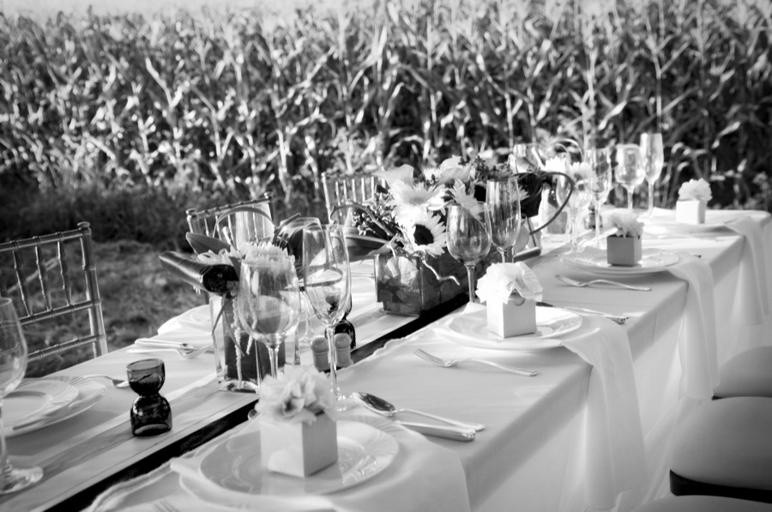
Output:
[351,390,487,434]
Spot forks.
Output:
[81,374,134,390]
[412,348,540,380]
[127,346,211,360]
[556,272,653,295]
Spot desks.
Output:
[81,207,771,512]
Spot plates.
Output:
[449,303,585,341]
[202,419,398,497]
[0,372,77,430]
[563,245,683,270]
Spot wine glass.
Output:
[238,203,361,420]
[446,178,523,302]
[557,133,664,245]
[1,298,45,495]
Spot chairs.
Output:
[322,171,388,241]
[186,191,273,294]
[638,495,772,512]
[711,345,771,397]
[668,396,771,501]
[2,221,109,375]
